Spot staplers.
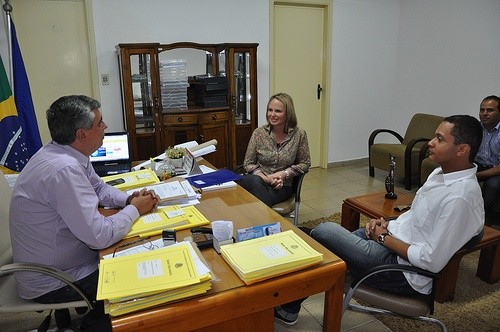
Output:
[191,227,236,249]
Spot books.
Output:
[90,168,322,317]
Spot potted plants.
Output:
[164,145,186,167]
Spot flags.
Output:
[0,0,43,173]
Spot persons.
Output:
[274,95,499,324]
[8,95,160,332]
[236,93,311,208]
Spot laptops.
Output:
[90,133,132,178]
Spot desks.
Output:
[95,157,347,332]
[340,188,500,304]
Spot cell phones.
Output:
[106,178,125,186]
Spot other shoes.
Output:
[274,305,299,328]
[80,315,113,332]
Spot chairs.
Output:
[341,228,485,332]
[368,113,445,191]
[0,168,95,332]
[417,141,440,189]
[231,164,311,227]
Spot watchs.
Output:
[377,233,388,243]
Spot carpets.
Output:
[295,212,500,332]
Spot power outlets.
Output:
[101,74,109,86]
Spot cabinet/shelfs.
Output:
[115,42,259,174]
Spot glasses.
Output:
[112,236,159,258]
